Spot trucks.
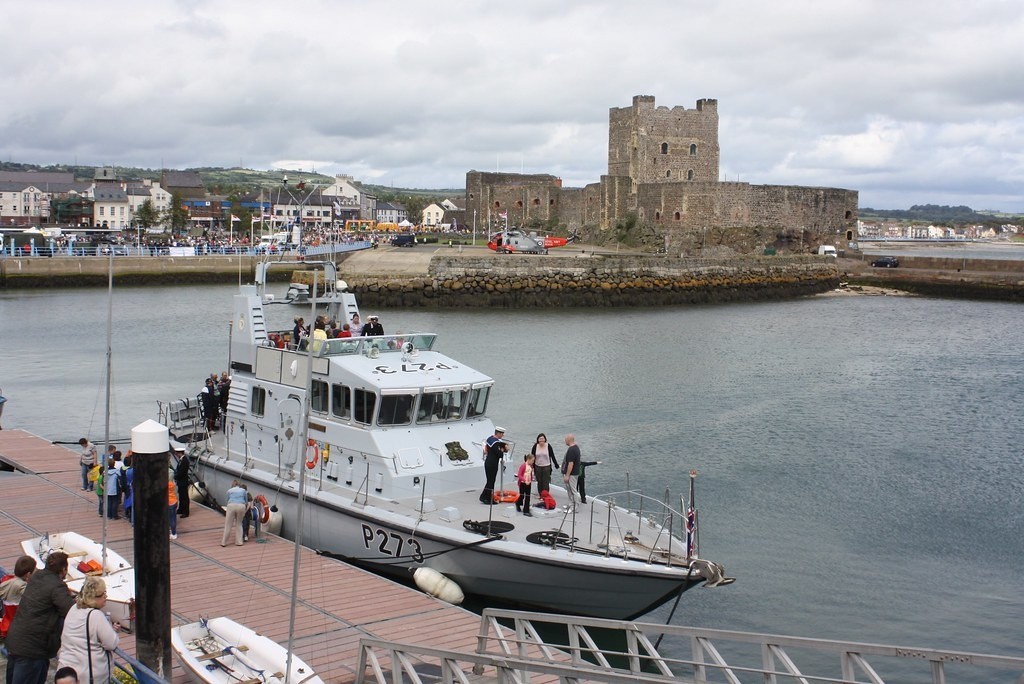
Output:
[817,245,838,258]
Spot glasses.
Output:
[96,591,107,598]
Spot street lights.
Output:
[702,226,707,251]
[800,226,804,254]
[835,229,840,251]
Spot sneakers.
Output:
[563,505,570,509]
[564,509,578,513]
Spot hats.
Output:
[174,448,185,452]
[495,426,506,432]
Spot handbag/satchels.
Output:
[90,465,100,482]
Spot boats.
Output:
[158,171,739,630]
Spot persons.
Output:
[174,450,190,518]
[562,435,580,513]
[532,490,556,511]
[515,454,535,516]
[169,455,178,539]
[57,577,121,684]
[531,433,559,500]
[221,480,253,547]
[201,372,231,435]
[55,667,80,684]
[5,552,78,684]
[293,314,384,353]
[0,556,37,656]
[479,427,508,504]
[578,461,602,504]
[79,438,97,491]
[3,225,283,255]
[338,388,436,422]
[96,445,132,522]
[389,331,403,348]
[301,227,486,249]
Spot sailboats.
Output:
[18,252,137,633]
[170,266,332,684]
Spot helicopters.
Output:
[486,223,583,254]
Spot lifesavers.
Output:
[493,491,520,502]
[306,438,319,469]
[253,495,270,524]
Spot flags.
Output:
[232,215,240,221]
[499,212,507,218]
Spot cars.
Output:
[871,256,899,269]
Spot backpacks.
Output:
[116,465,131,492]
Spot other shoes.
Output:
[171,533,177,539]
[516,503,521,511]
[175,510,182,514]
[523,512,532,516]
[244,535,248,541]
[180,514,189,518]
[480,498,498,504]
